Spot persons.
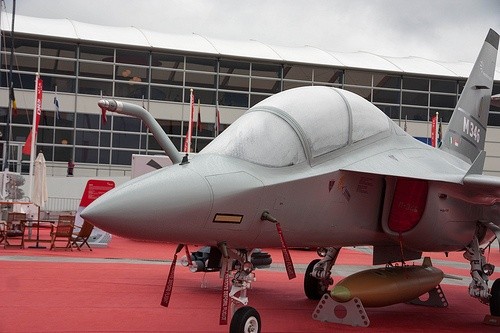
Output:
[67,160,74,176]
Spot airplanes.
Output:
[80,27,500,333]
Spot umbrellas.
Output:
[32,150,47,247]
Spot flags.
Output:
[11,78,60,154]
[184,94,220,152]
[431,117,436,147]
[102,109,107,124]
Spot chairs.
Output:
[66,219,107,251]
[49,215,75,251]
[0,212,28,248]
